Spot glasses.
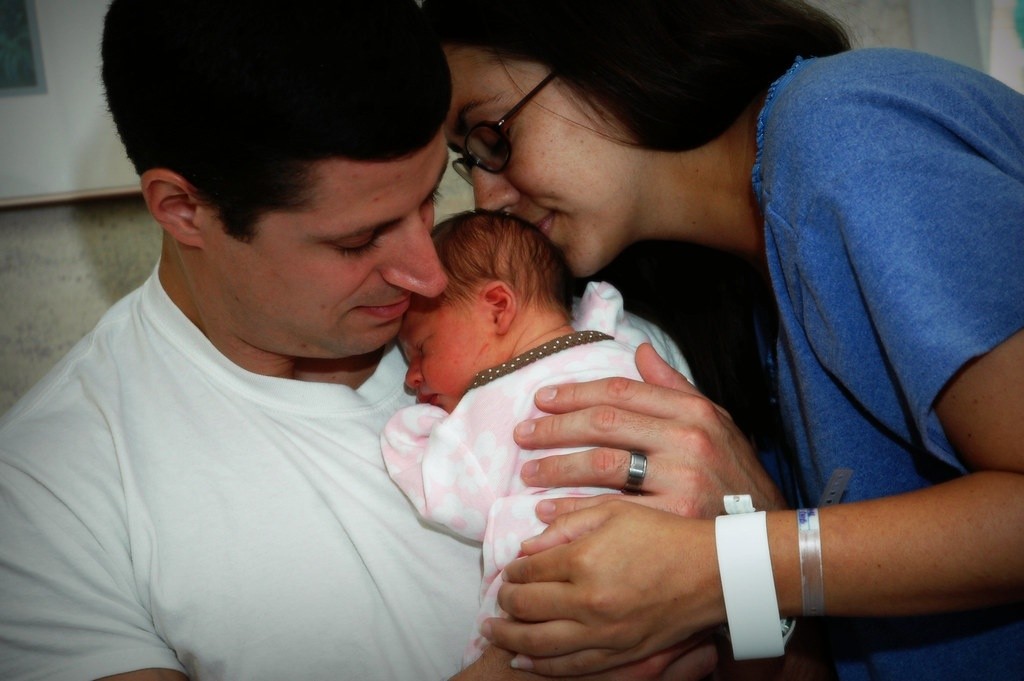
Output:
[451,72,556,186]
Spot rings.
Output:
[620,449,647,495]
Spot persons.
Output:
[0,1,789,681]
[381,209,697,671]
[421,0,1024,680]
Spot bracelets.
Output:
[714,493,796,662]
[796,508,825,617]
